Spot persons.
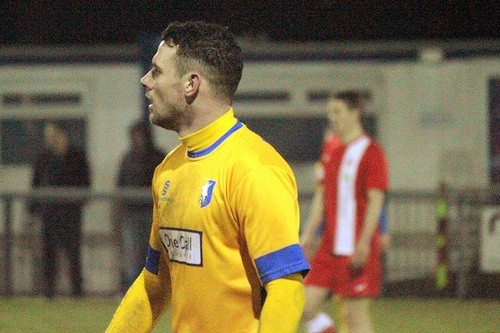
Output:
[295,86,376,252]
[109,119,168,299]
[28,121,94,300]
[100,19,310,333]
[295,90,391,333]
